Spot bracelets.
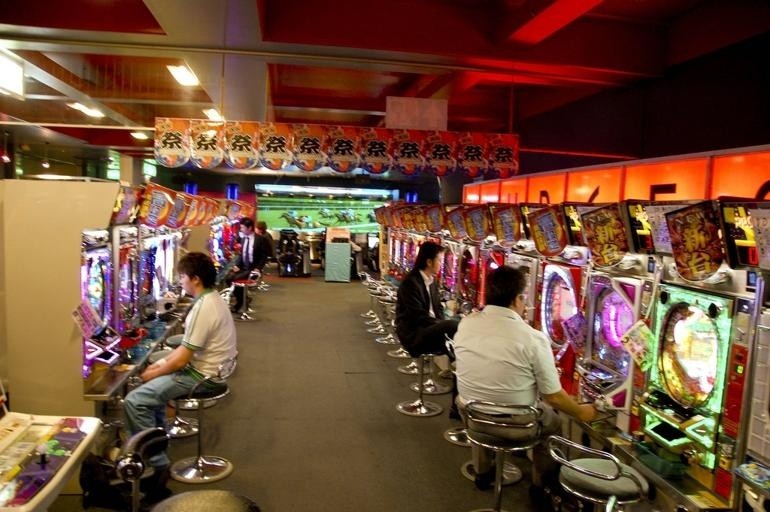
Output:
[156,358,166,366]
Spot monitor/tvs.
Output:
[78,219,240,337]
[385,223,732,418]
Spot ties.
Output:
[245,237,249,268]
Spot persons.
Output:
[123,252,239,508]
[394,244,459,423]
[255,222,276,261]
[225,217,267,311]
[452,267,597,494]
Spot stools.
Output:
[360,269,453,417]
[444,332,477,448]
[458,398,543,512]
[548,435,651,512]
[114,334,261,512]
[232,270,261,322]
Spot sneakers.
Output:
[450,406,457,418]
[475,465,495,490]
